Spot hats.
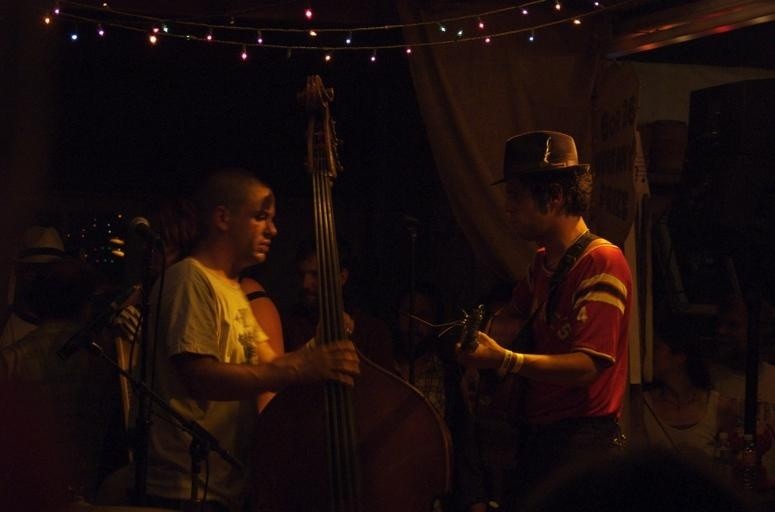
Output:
[6,223,71,265]
[489,130,592,187]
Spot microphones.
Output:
[131,216,165,256]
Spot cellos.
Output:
[249,74,452,512]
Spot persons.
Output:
[142,165,359,512]
[641,291,774,512]
[456,130,632,512]
[282,227,490,512]
[2,226,118,505]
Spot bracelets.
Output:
[496,350,525,375]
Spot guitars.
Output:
[464,306,529,468]
